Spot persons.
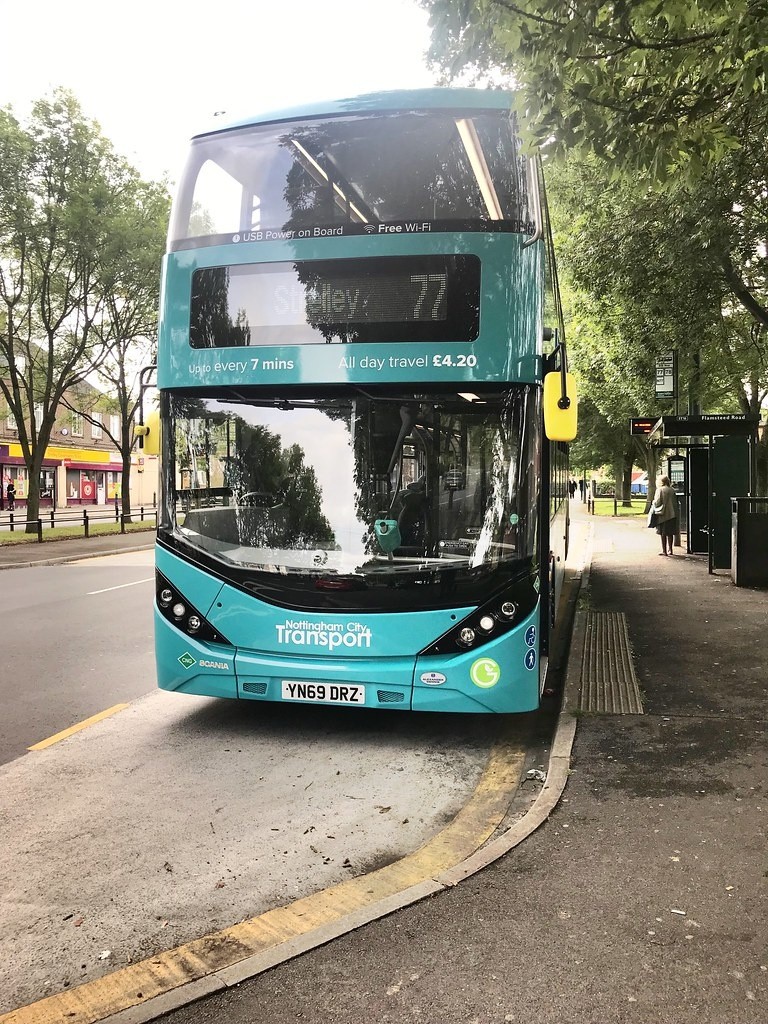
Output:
[652,476,679,556]
[5,479,16,511]
[569,478,588,499]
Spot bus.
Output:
[133,86,579,712]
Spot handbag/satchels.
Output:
[647,502,657,528]
[655,488,665,515]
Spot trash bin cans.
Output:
[730,497,768,588]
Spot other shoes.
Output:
[659,552,667,556]
[667,550,672,555]
